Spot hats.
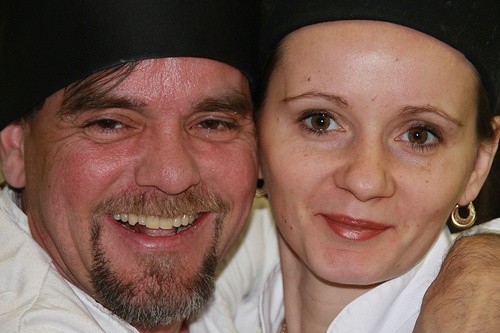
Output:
[0,0,259,133]
[259,0,500,114]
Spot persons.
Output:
[258,0,500,333]
[0,0,500,333]
